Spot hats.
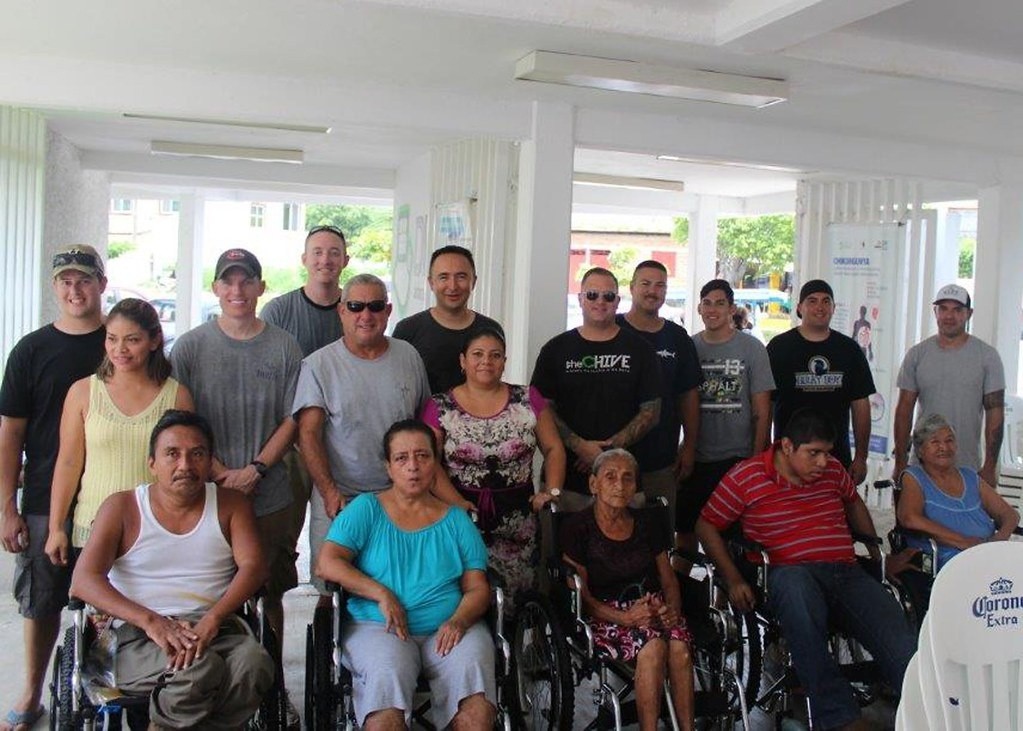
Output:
[51,245,104,280]
[214,248,261,282]
[797,279,835,319]
[933,285,971,311]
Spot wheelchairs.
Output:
[730,532,917,731]
[305,580,575,731]
[543,544,752,731]
[50,598,301,731]
[874,479,1023,629]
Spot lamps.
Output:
[146,138,307,165]
[507,51,795,114]
[572,173,686,193]
[125,114,326,136]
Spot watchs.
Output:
[251,461,268,479]
[546,488,562,497]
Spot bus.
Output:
[666,287,792,327]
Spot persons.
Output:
[44,299,197,566]
[0,245,113,731]
[674,279,776,609]
[163,249,305,731]
[315,419,498,731]
[71,409,273,731]
[897,414,1020,571]
[391,246,505,395]
[892,284,1005,485]
[290,274,433,608]
[616,261,704,514]
[765,279,877,484]
[529,267,663,513]
[555,448,696,731]
[256,225,349,356]
[697,408,916,731]
[420,325,565,639]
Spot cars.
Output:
[141,298,220,348]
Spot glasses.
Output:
[52,253,102,272]
[307,225,346,248]
[580,292,618,302]
[342,300,389,313]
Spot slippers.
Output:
[0,702,45,731]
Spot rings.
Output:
[667,620,670,625]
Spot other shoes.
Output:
[524,642,550,675]
[276,691,300,729]
